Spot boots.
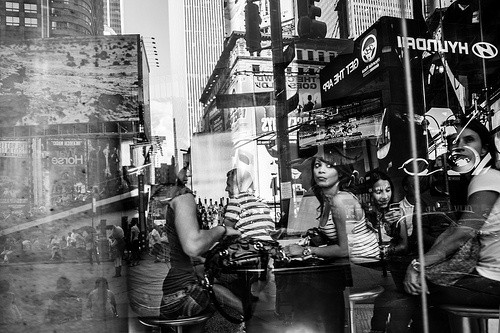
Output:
[111,266,120,278]
[119,264,122,276]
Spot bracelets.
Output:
[218,223,227,237]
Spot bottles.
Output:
[196,196,229,230]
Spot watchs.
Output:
[302,245,311,257]
[411,260,421,272]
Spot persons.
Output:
[44,276,83,324]
[354,168,437,261]
[281,153,380,294]
[0,220,140,278]
[402,116,500,310]
[0,280,22,325]
[201,168,276,333]
[85,277,118,320]
[127,153,241,320]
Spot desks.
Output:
[224,255,379,333]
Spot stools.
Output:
[139,308,214,333]
[347,286,384,332]
[440,305,500,333]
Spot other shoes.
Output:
[90,261,93,264]
[254,309,283,323]
[97,261,100,265]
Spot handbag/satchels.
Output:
[424,235,480,287]
[302,227,329,247]
[204,273,244,325]
[350,260,397,292]
